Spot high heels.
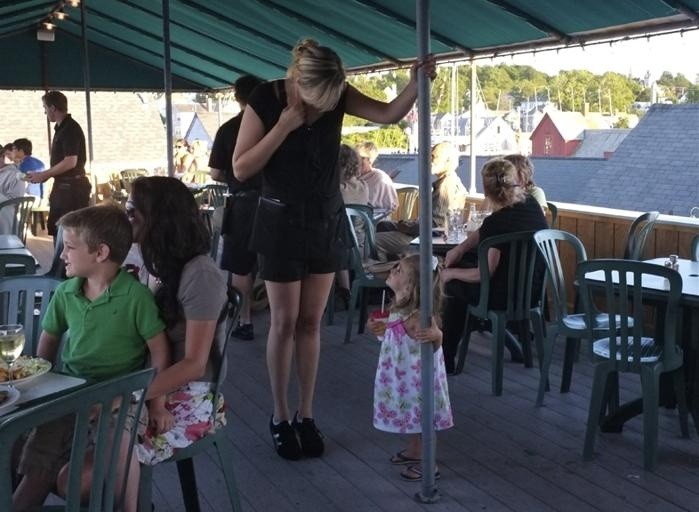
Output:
[339,287,360,311]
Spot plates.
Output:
[0,357,55,386]
[0,387,28,410]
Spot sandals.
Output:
[389,448,441,482]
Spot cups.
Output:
[445,209,466,242]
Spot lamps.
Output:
[40,3,70,31]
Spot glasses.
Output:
[124,200,138,218]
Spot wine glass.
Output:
[0,323,29,393]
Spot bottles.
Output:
[466,203,481,237]
[664,255,680,279]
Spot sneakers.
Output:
[268,408,325,461]
[231,321,255,341]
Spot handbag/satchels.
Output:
[249,195,288,259]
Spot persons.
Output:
[372,139,471,270]
[438,156,552,378]
[336,139,372,311]
[1,89,199,264]
[355,141,401,229]
[231,34,441,460]
[57,171,233,511]
[363,255,455,483]
[1,200,175,511]
[206,72,267,343]
[502,153,547,218]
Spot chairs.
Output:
[581,211,696,441]
[451,230,550,396]
[346,181,558,361]
[1,163,344,324]
[532,228,640,420]
[576,260,696,467]
[1,278,243,509]
